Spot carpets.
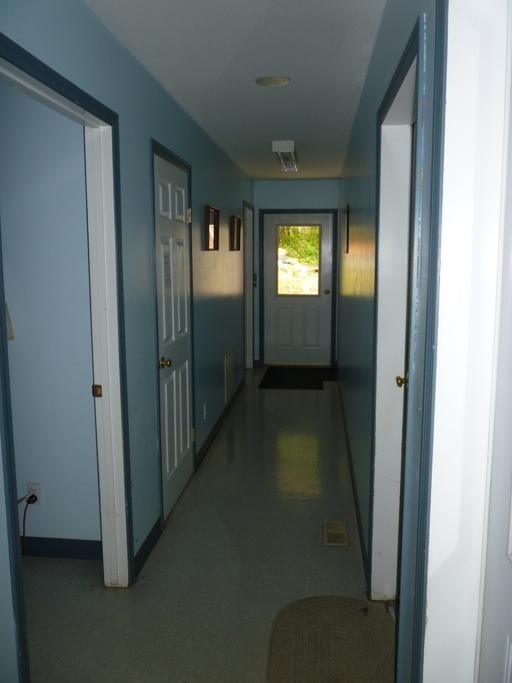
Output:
[267,595,395,683]
[258,366,337,389]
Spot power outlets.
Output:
[27,482,40,504]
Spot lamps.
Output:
[272,140,298,172]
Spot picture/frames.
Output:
[231,216,240,251]
[204,205,219,251]
